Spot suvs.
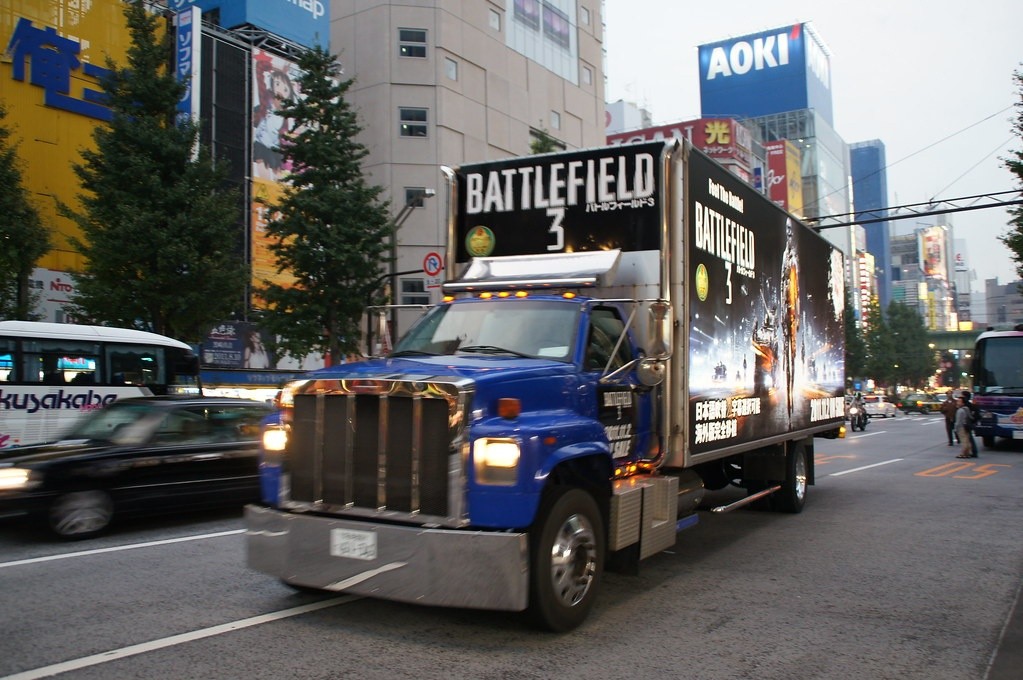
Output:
[862,395,897,418]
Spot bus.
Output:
[0,319,208,448]
[972,331,1023,448]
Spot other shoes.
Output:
[957,440,960,444]
[970,454,977,457]
[956,455,971,458]
[948,443,953,446]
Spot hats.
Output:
[945,390,953,394]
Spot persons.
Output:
[961,391,978,458]
[588,323,611,369]
[244,328,269,368]
[848,392,867,425]
[940,390,960,446]
[954,398,971,458]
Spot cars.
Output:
[898,391,944,414]
[845,395,857,415]
[935,394,952,403]
[0,394,275,542]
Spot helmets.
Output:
[854,391,861,398]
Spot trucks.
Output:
[246,140,847,634]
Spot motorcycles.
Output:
[848,404,867,431]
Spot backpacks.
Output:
[970,406,981,423]
[961,408,976,432]
